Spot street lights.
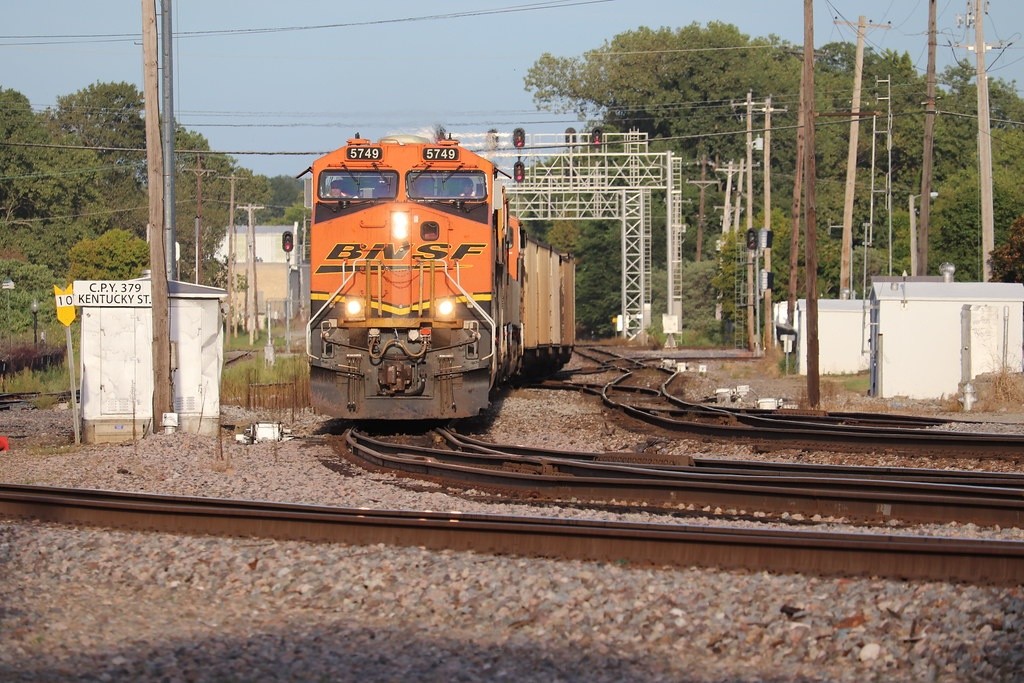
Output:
[30,299,39,349]
[909,191,939,277]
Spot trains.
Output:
[304,132,575,429]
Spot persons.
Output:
[327,180,350,197]
[458,178,477,197]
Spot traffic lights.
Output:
[514,161,525,182]
[592,127,602,148]
[747,228,758,250]
[282,231,294,253]
[513,127,525,148]
